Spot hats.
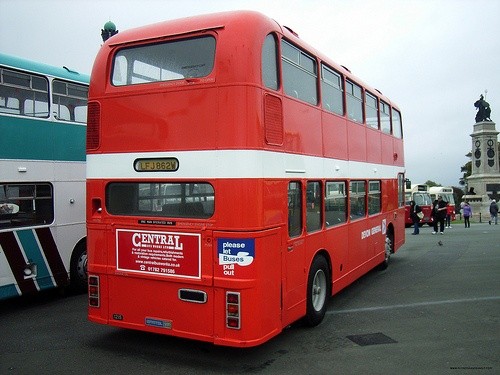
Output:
[438,196,443,199]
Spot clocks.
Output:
[487,139,494,146]
[475,140,480,146]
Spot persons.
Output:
[430,195,499,235]
[474,95,490,122]
[408,201,422,234]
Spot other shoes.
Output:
[431,231,437,235]
[439,231,444,235]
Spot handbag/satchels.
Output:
[414,205,425,219]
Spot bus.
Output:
[405,184,434,228]
[85,10,406,350]
[430,184,456,222]
[0,53,88,307]
